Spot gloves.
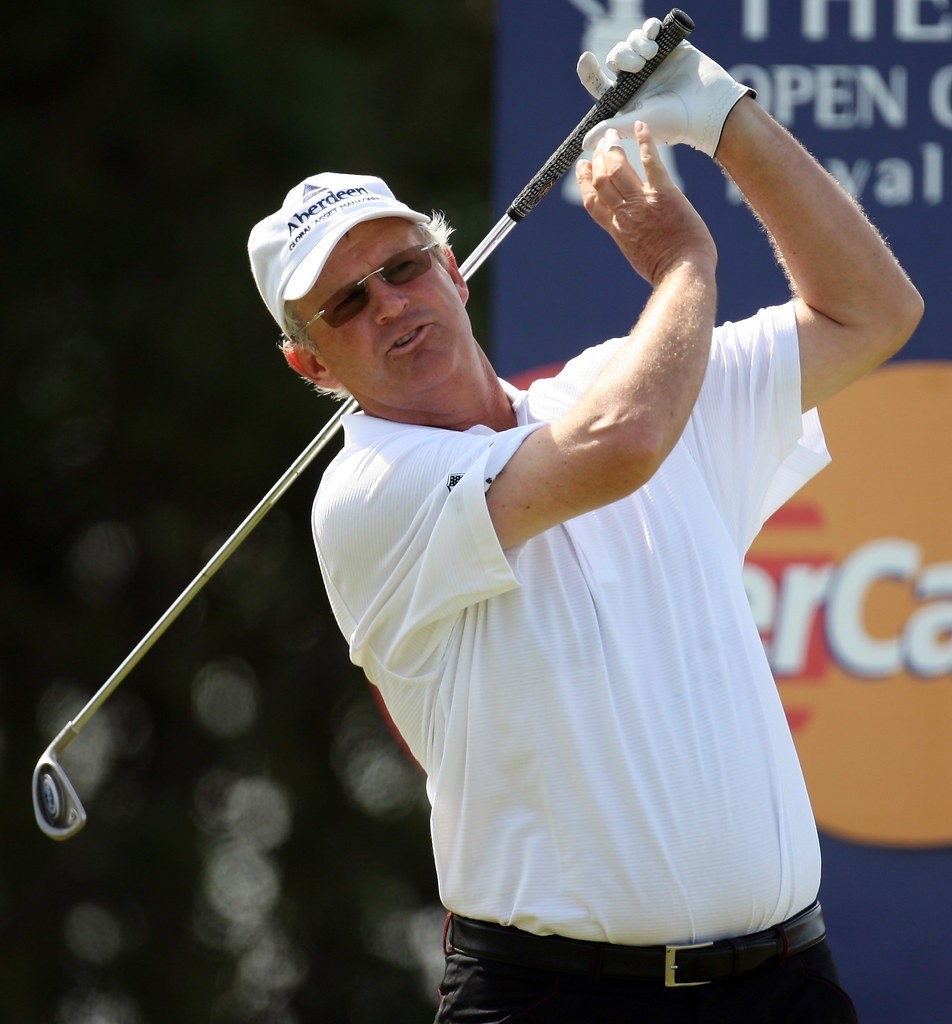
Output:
[575,16,757,159]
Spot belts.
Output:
[448,901,826,987]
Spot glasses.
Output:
[290,241,440,342]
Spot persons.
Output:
[248,17,925,1024]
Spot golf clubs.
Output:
[30,8,701,844]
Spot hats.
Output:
[247,171,430,342]
